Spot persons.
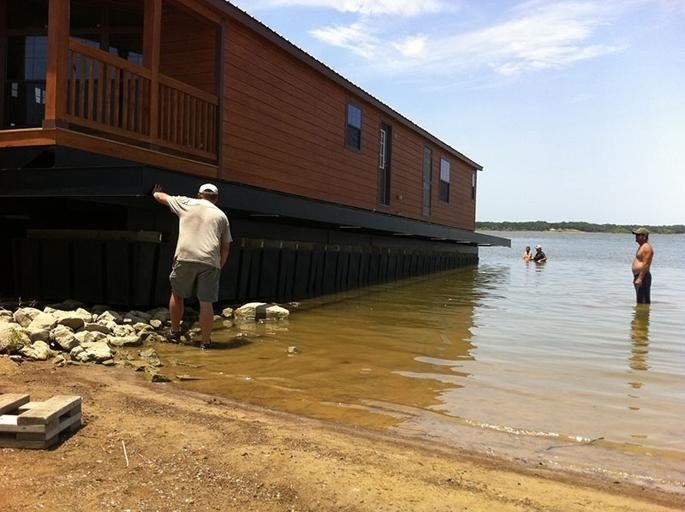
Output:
[523,246,531,262]
[534,245,547,264]
[151,182,233,350]
[631,228,654,304]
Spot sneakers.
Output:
[159,330,181,345]
[200,341,214,349]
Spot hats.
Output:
[631,227,650,235]
[535,244,543,250]
[197,182,219,197]
